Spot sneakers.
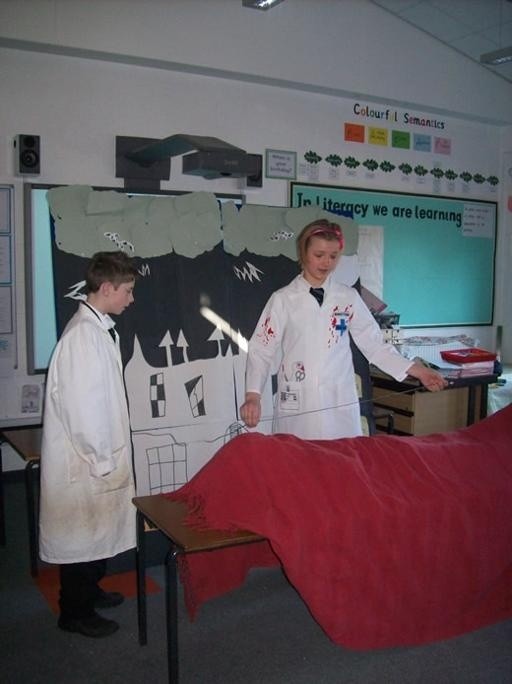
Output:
[58,590,124,637]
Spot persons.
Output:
[239,217,452,441]
[38,249,139,639]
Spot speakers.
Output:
[15,134,42,178]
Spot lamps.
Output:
[480,1,512,65]
[242,0,284,12]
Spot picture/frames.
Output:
[266,148,297,180]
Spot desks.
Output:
[1,427,42,577]
[132,496,266,684]
[370,368,497,436]
